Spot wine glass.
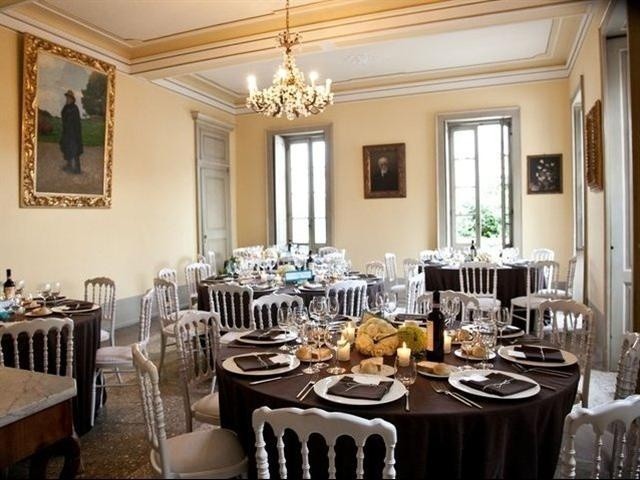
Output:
[0,267,68,318]
[275,289,524,386]
[231,240,523,280]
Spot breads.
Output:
[457,330,469,341]
[416,361,442,375]
[296,347,311,360]
[359,356,384,374]
[312,348,330,359]
[472,343,486,357]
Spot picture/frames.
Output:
[583,100,602,193]
[361,142,407,199]
[526,153,565,196]
[16,31,118,209]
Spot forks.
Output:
[430,381,482,409]
[512,362,574,377]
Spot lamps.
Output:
[242,1,338,122]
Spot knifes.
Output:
[249,373,304,386]
[405,388,412,412]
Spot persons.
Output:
[58,89,89,174]
[375,158,398,189]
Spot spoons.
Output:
[295,373,319,399]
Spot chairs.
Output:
[85,277,125,387]
[1,317,87,475]
[91,287,154,431]
[153,244,640,479]
[132,344,249,479]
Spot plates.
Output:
[447,370,541,401]
[497,345,578,367]
[222,352,301,377]
[312,373,407,407]
[235,330,298,345]
[50,303,100,313]
[203,277,234,283]
